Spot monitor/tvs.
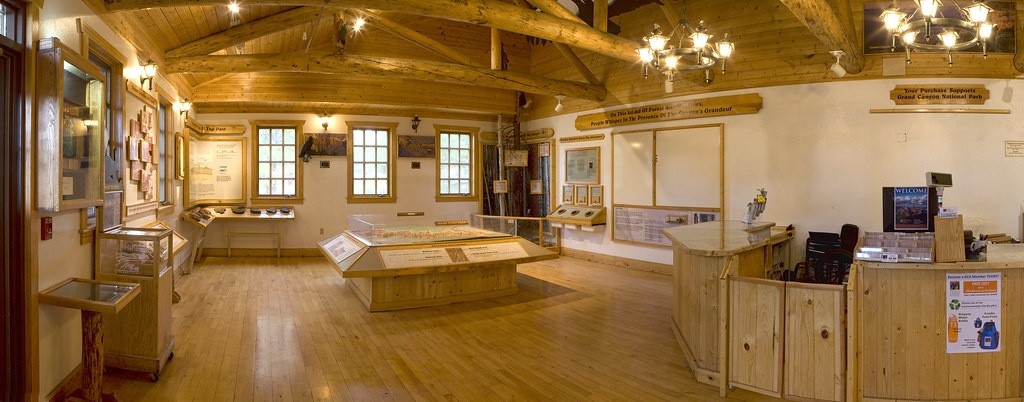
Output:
[883,186,939,233]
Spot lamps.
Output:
[831,50,847,77]
[322,110,328,130]
[555,96,566,111]
[139,58,157,90]
[635,0,734,86]
[878,0,998,68]
[411,113,421,133]
